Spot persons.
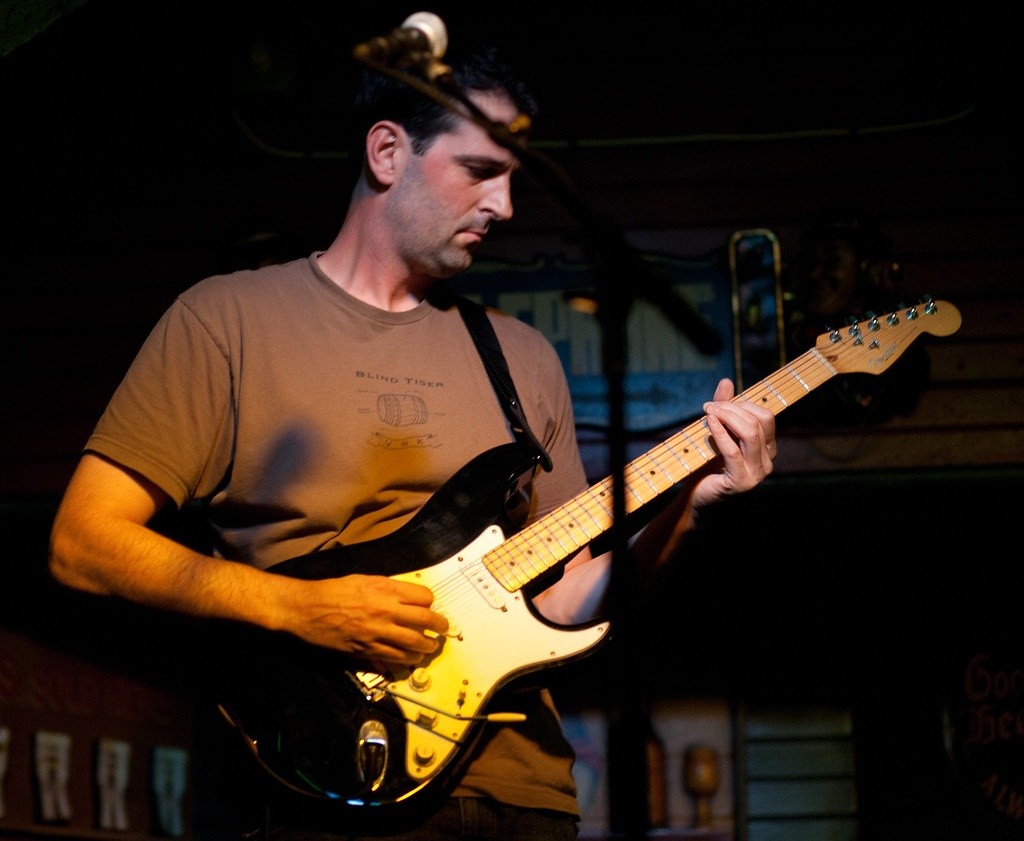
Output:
[47,56,780,841]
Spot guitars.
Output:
[200,295,964,836]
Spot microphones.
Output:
[354,11,449,63]
